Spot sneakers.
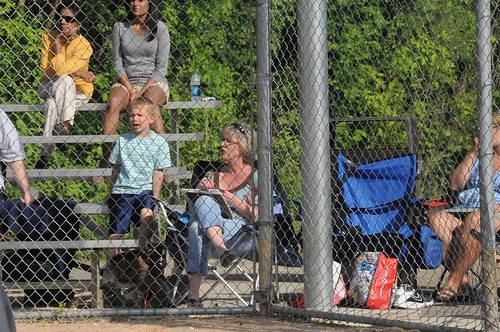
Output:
[391,283,433,309]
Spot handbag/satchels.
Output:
[346,250,398,311]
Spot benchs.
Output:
[0,100,223,309]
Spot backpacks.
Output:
[99,245,165,309]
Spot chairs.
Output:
[146,160,281,308]
[291,153,429,295]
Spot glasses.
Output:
[231,122,248,146]
[56,16,76,23]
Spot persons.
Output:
[108,97,171,274]
[428,104,500,302]
[34,2,96,170]
[92,0,175,183]
[177,123,283,309]
[0,109,34,205]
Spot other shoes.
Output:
[220,249,236,267]
[178,298,203,308]
[35,157,49,169]
[435,289,458,302]
[52,124,69,151]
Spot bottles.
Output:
[191,71,201,101]
